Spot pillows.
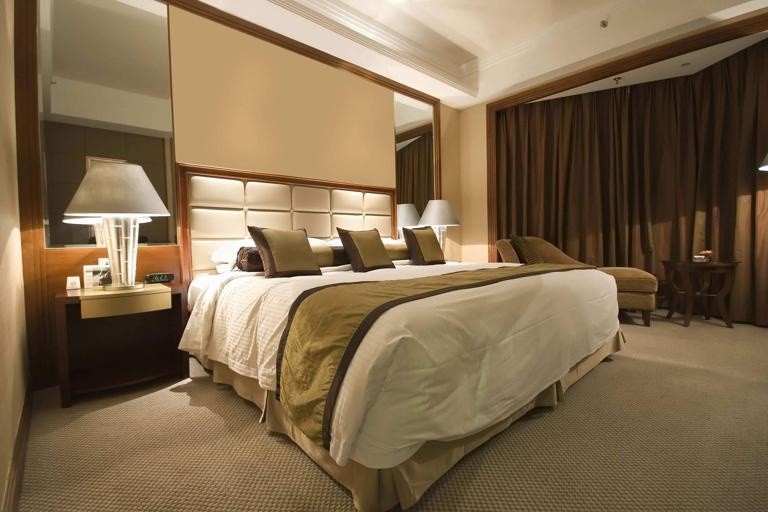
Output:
[244,224,323,279]
[508,236,544,265]
[334,226,396,273]
[401,225,445,266]
[233,241,414,273]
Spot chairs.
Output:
[494,235,658,330]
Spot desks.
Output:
[660,258,742,331]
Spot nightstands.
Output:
[55,280,191,409]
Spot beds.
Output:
[178,161,629,512]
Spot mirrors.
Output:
[393,90,437,241]
[32,1,185,251]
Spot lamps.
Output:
[63,215,151,245]
[758,153,768,172]
[65,160,172,289]
[416,199,462,259]
[395,204,422,240]
